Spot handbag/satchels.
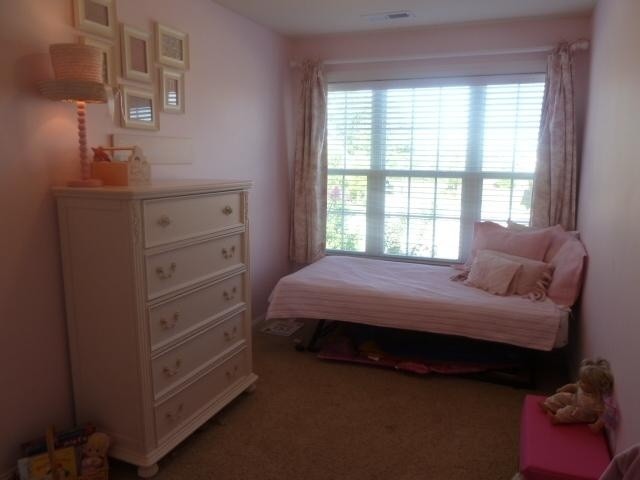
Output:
[1,424,110,480]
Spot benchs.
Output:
[518,390,615,480]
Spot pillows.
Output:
[458,219,588,312]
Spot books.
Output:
[17,423,91,480]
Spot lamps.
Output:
[28,40,113,181]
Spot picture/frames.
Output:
[78,35,117,100]
[72,0,118,41]
[120,22,155,84]
[158,67,187,116]
[118,82,161,132]
[155,22,191,72]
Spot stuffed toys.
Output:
[544,358,620,435]
[80,433,113,480]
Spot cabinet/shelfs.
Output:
[51,179,263,476]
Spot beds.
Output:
[265,218,589,386]
